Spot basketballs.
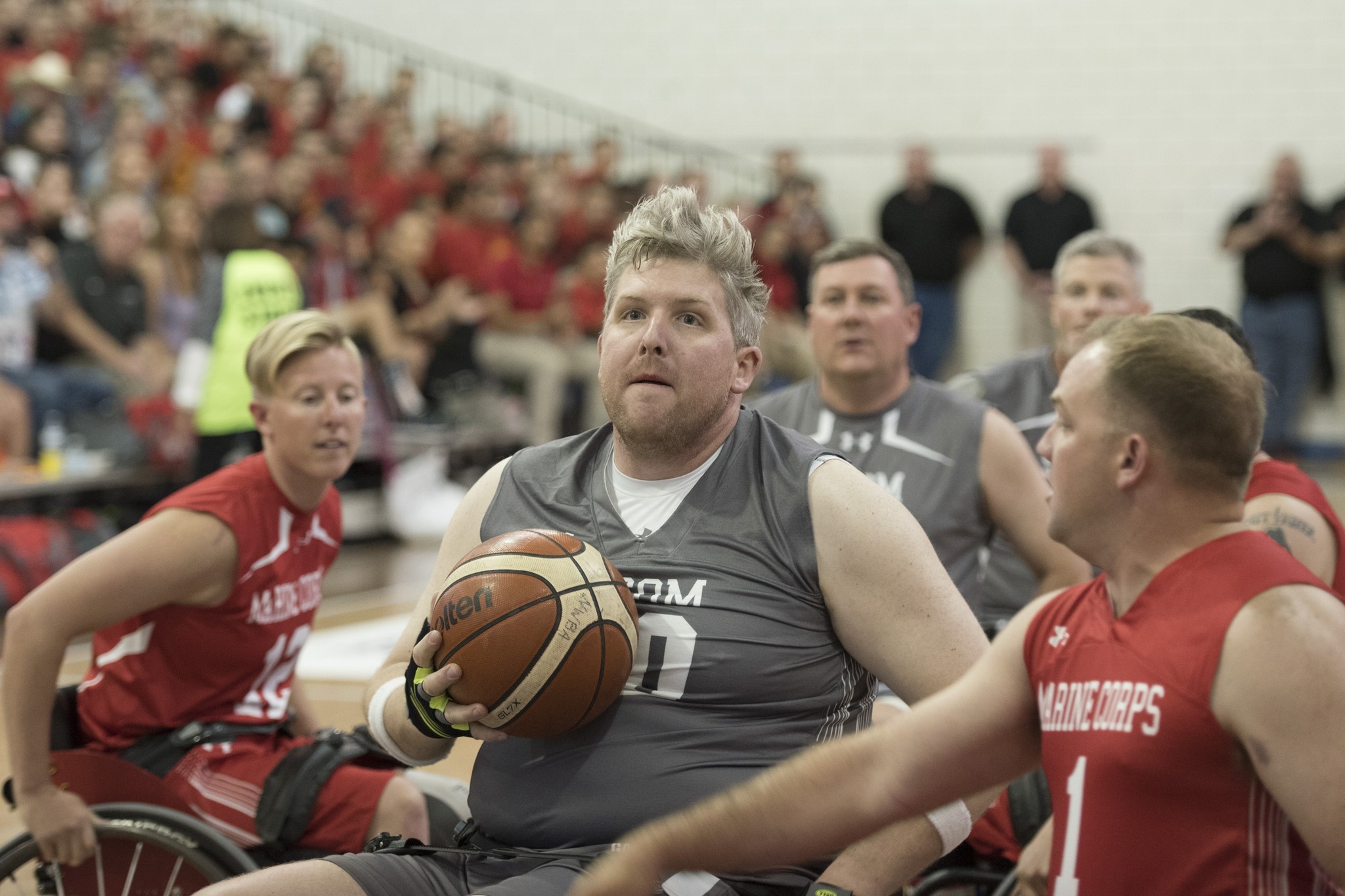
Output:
[427,528,640,742]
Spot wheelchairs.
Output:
[0,682,262,896]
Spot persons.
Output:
[189,181,1007,896]
[3,306,468,868]
[565,312,1345,896]
[874,135,985,377]
[0,1,1345,896]
[744,233,1094,722]
[1219,148,1342,459]
[1004,146,1101,353]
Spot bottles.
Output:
[38,410,66,477]
